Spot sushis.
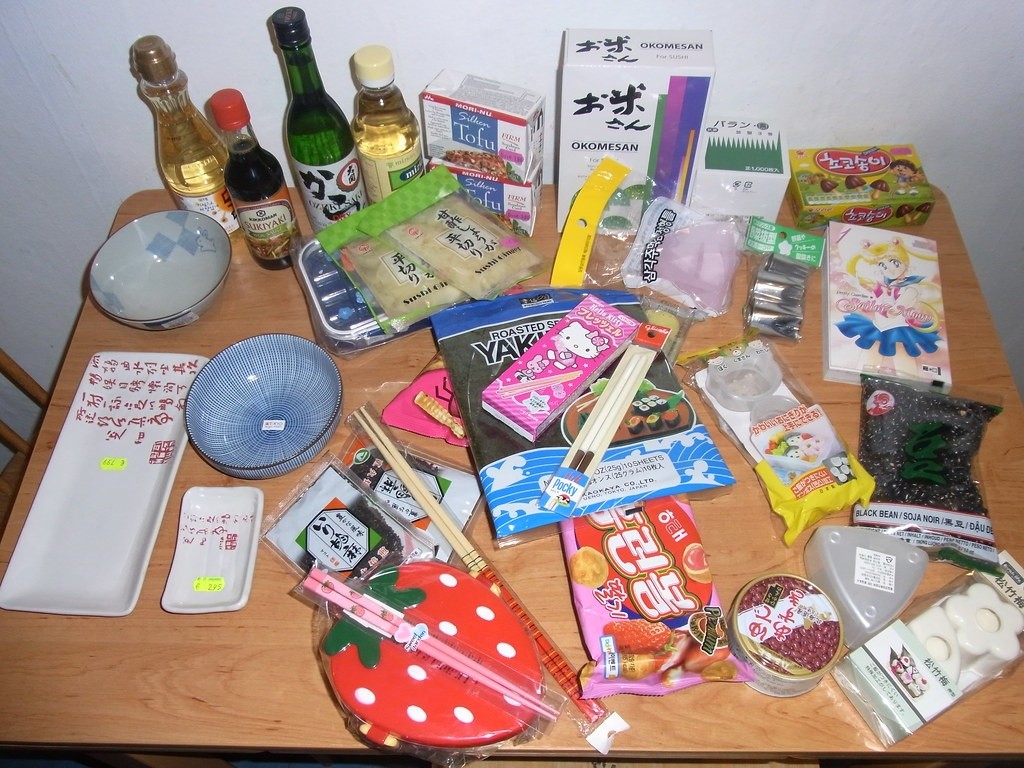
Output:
[627,394,681,433]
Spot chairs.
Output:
[0,348,52,550]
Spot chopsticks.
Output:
[567,351,648,474]
[304,568,560,722]
[352,406,605,723]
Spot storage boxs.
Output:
[830,550,1024,748]
[687,118,792,225]
[786,145,936,232]
[557,29,717,235]
[420,68,545,237]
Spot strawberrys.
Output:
[604,618,732,676]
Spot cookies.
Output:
[820,175,932,225]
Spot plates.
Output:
[0,351,210,617]
[160,485,264,615]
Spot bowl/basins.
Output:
[184,333,344,480]
[88,209,233,331]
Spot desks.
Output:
[1,184,1024,768]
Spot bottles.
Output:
[210,89,306,271]
[132,35,240,234]
[270,6,369,234]
[351,45,426,207]
